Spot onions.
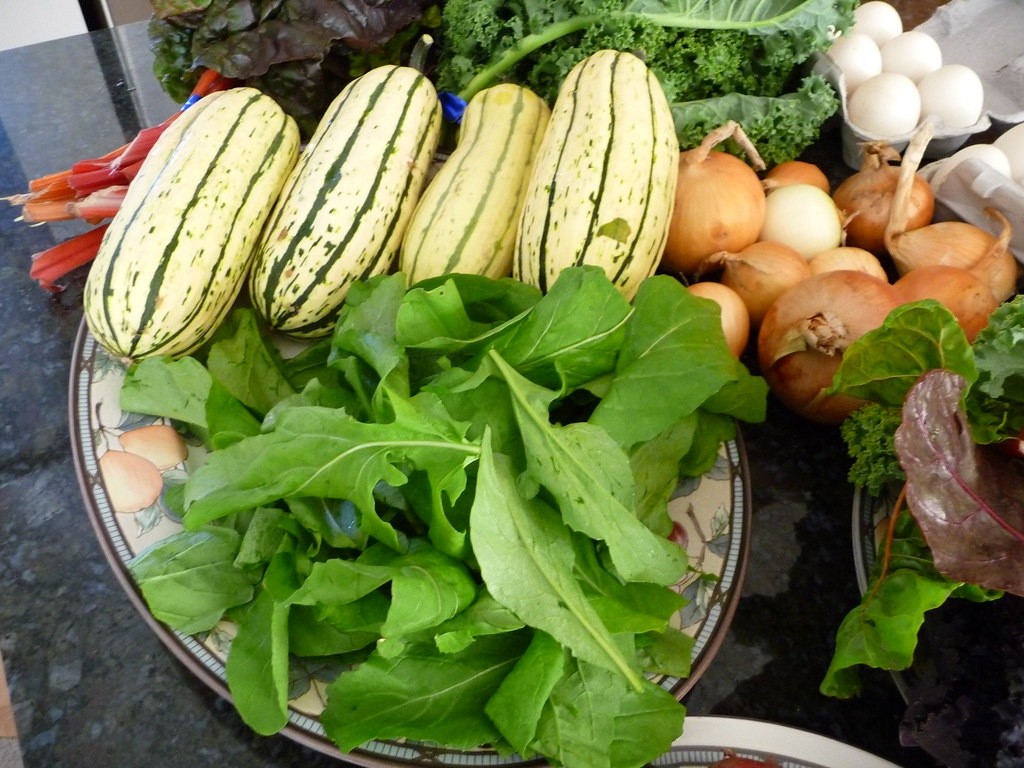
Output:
[660,119,1019,425]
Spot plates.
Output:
[853,485,915,705]
[643,715,900,768]
[68,143,752,768]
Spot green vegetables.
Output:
[105,264,768,768]
[818,292,1024,768]
[145,0,859,163]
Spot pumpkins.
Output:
[84,47,679,363]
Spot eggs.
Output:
[822,0,1024,194]
